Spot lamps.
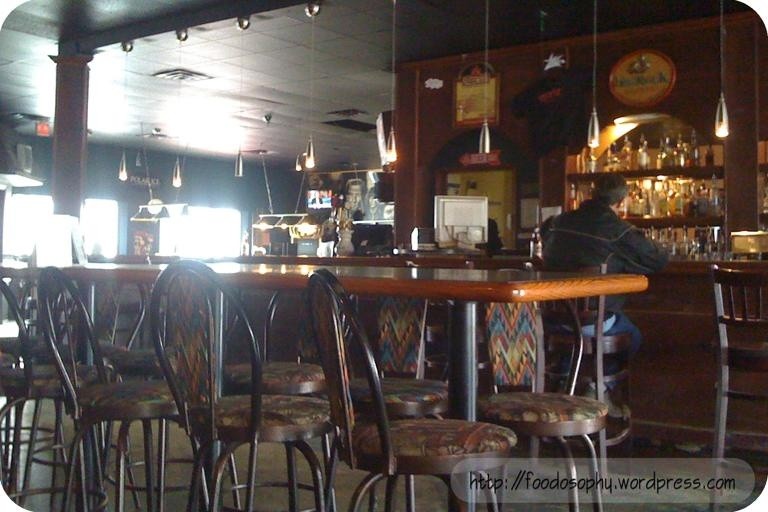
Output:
[118,42,132,181]
[172,32,189,188]
[136,151,143,166]
[478,0,490,154]
[587,0,600,149]
[305,4,321,169]
[715,0,730,138]
[234,17,250,178]
[130,203,189,221]
[251,213,318,235]
[386,0,398,162]
[295,155,303,172]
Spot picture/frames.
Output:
[450,71,502,129]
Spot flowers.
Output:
[330,195,355,231]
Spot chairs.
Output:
[0,261,605,512]
[706,263,768,512]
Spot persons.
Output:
[344,178,366,216]
[540,171,668,420]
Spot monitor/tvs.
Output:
[434,195,489,250]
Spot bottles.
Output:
[528,218,544,263]
[573,130,723,258]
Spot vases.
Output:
[333,229,355,257]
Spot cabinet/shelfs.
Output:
[566,164,725,233]
[757,159,768,227]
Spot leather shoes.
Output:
[581,385,624,419]
